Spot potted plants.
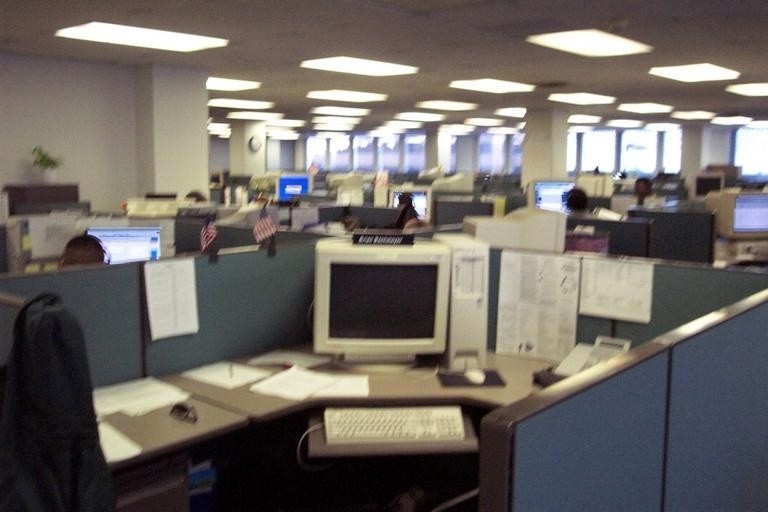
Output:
[33,144,64,186]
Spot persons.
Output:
[634,178,653,205]
[566,187,589,218]
[398,193,419,219]
[58,230,108,273]
[0,292,116,512]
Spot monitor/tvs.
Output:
[389,185,432,221]
[705,189,768,240]
[85,227,161,266]
[330,175,361,192]
[276,176,312,201]
[685,174,725,199]
[462,207,567,253]
[314,236,452,372]
[432,171,474,193]
[527,178,576,214]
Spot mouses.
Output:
[464,369,486,385]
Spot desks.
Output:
[163,345,567,512]
[90,376,249,512]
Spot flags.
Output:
[253,214,278,243]
[200,220,218,254]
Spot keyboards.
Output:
[324,405,466,445]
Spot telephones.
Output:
[553,335,633,378]
